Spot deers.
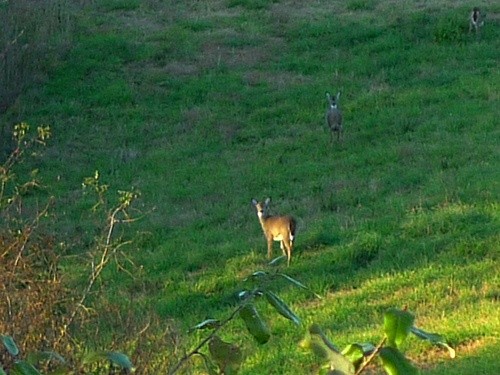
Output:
[252,197,296,266]
[325,91,343,144]
[469,6,487,34]
